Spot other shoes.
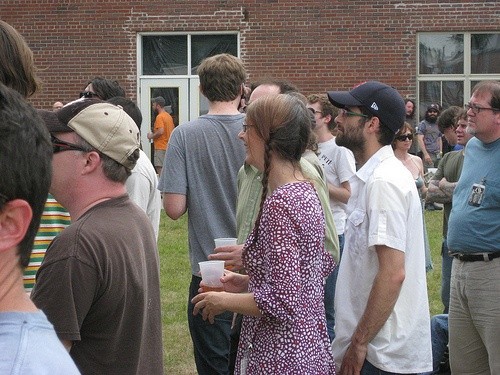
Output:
[425,202,443,210]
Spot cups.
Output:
[198,260,225,292]
[214,237,238,270]
[427,168,438,180]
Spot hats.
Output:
[152,96,165,105]
[428,103,439,113]
[326,81,406,135]
[34,96,141,172]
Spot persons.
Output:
[329,81,433,375]
[36,98,164,375]
[210,76,356,340]
[0,85,80,375]
[447,80,500,375]
[393,98,474,314]
[191,95,335,375]
[0,20,161,298]
[147,97,174,198]
[158,54,247,375]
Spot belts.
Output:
[448,249,500,262]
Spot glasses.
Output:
[53,106,61,109]
[464,103,500,114]
[396,133,413,141]
[79,92,98,98]
[337,108,369,119]
[242,121,254,132]
[429,110,437,113]
[307,108,322,114]
[438,127,447,134]
[50,132,106,160]
